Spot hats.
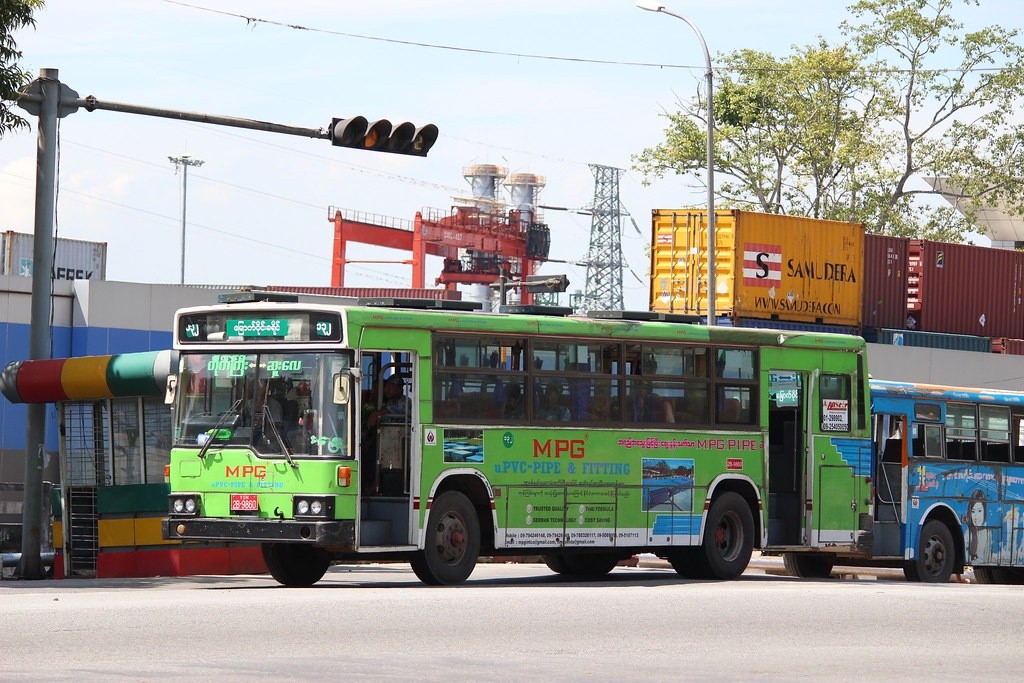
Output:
[383,374,404,386]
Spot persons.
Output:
[630,371,676,425]
[965,489,993,564]
[366,375,412,495]
[536,378,571,422]
[244,375,284,450]
[495,382,526,420]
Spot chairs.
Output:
[296,381,739,436]
[872,438,1024,462]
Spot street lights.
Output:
[639,3,717,324]
[168,154,204,285]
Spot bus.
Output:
[771,371,1023,589]
[166,288,875,586]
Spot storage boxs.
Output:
[648,207,1024,355]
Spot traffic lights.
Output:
[331,116,441,157]
